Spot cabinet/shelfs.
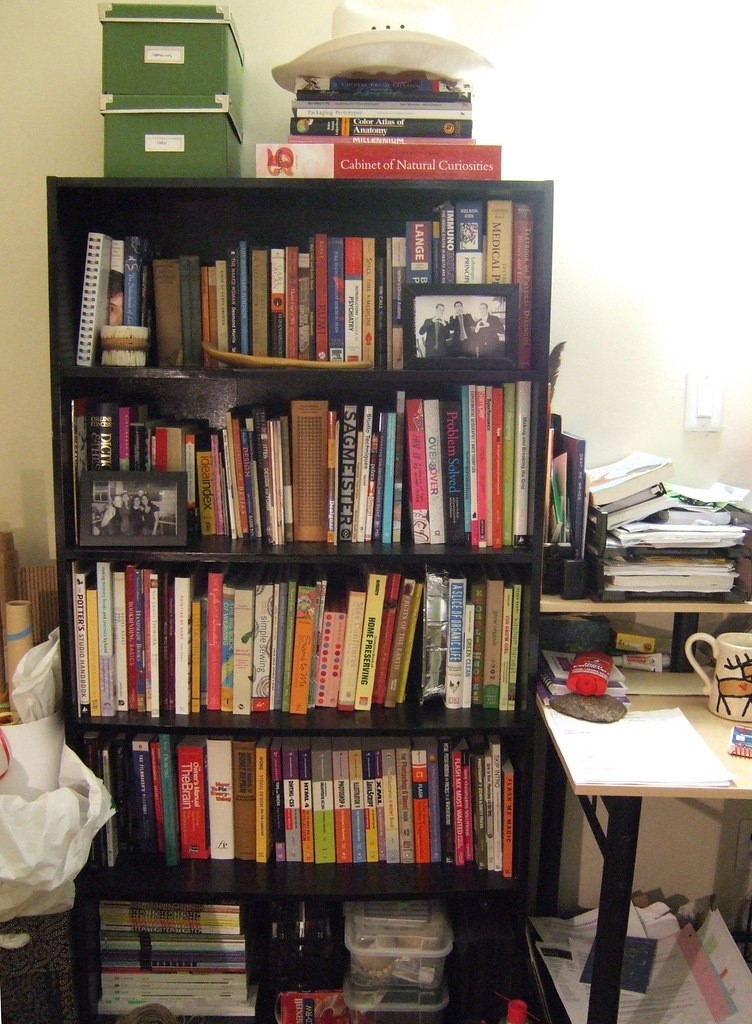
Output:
[45,172,554,1024]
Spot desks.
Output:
[529,668,752,1024]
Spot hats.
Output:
[271,0,495,94]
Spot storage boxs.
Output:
[342,902,454,988]
[98,2,246,129]
[99,94,242,179]
[343,978,450,1024]
[274,989,375,1024]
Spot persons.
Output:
[101,494,123,535]
[446,300,477,355]
[420,303,449,356]
[129,489,147,512]
[114,492,136,534]
[129,496,144,535]
[473,304,505,354]
[138,494,160,534]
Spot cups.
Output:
[685,632,752,723]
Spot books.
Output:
[67,199,731,1024]
[289,73,474,142]
